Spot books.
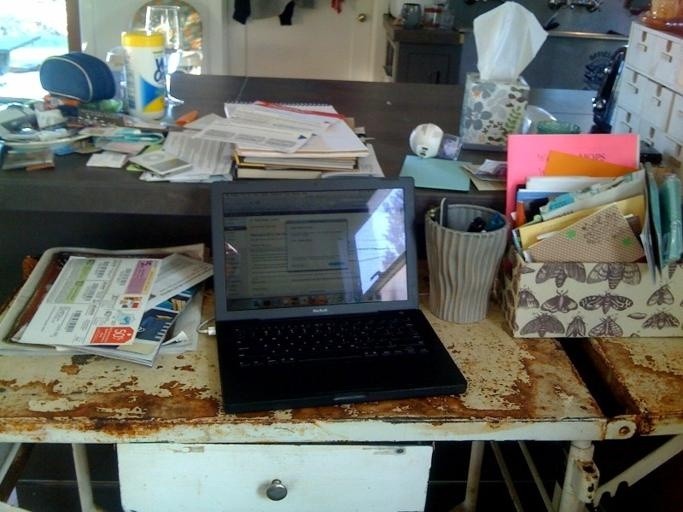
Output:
[51,285,194,368]
[507,133,683,285]
[223,100,371,180]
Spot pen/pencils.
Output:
[430,209,437,222]
[469,213,504,232]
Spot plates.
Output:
[0,135,90,152]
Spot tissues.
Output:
[460,3,549,154]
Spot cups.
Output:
[422,5,442,31]
[425,205,509,322]
[534,120,583,136]
[402,3,420,30]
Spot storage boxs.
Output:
[493,238,681,337]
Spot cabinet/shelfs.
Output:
[608,22,682,159]
[377,12,461,83]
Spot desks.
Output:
[587,335,682,512]
[0,76,246,229]
[0,257,636,512]
[0,31,40,75]
[200,74,659,218]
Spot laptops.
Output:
[212,176,468,414]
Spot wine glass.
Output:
[143,6,185,111]
